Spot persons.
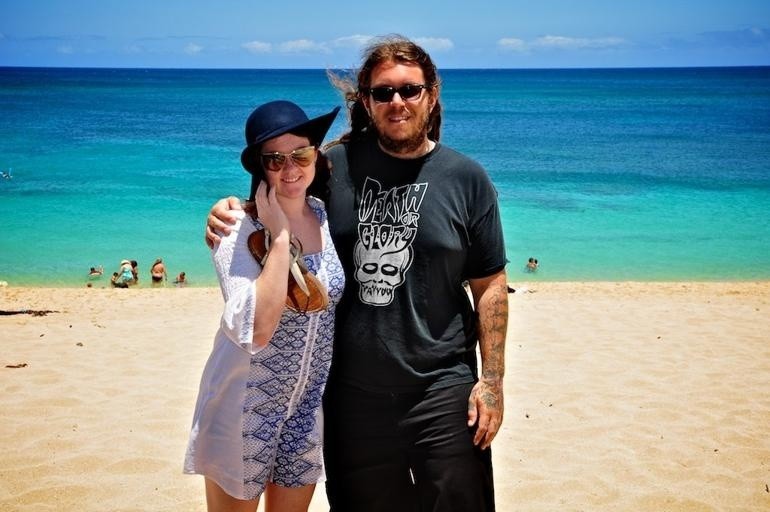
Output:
[205,40,507,512]
[535,259,538,266]
[526,258,537,273]
[183,100,346,512]
[87,259,186,286]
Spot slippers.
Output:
[247,227,330,313]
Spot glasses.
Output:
[365,83,428,103]
[262,146,316,173]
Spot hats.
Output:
[240,100,341,174]
